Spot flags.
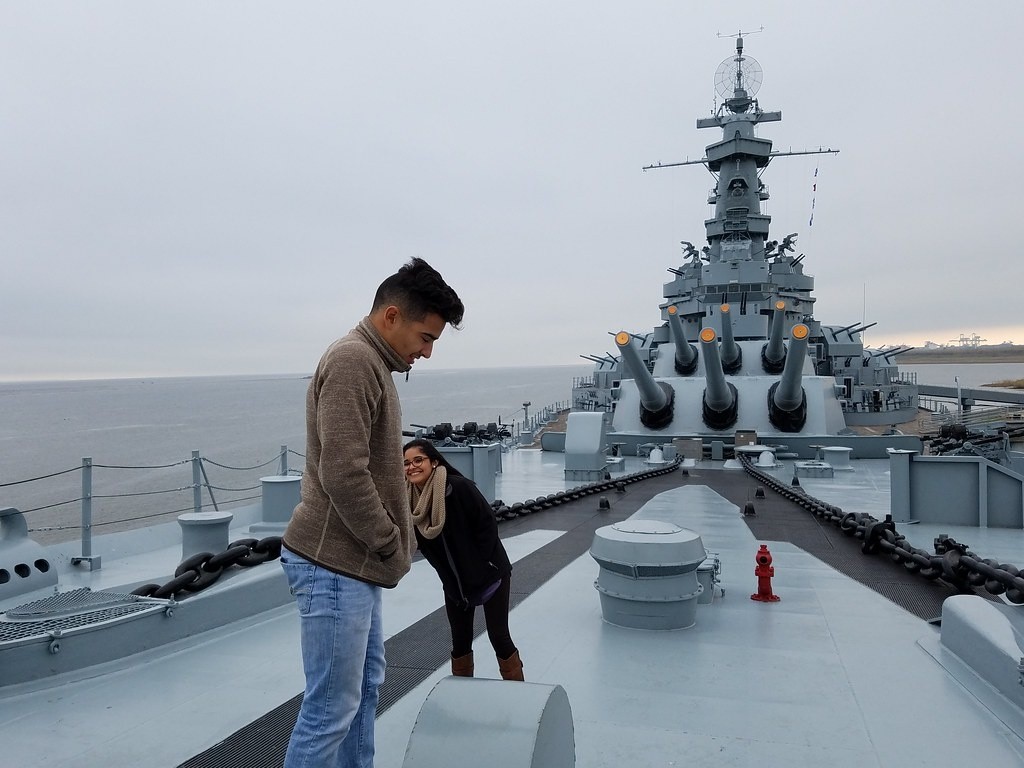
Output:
[810,168,818,226]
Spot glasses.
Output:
[402,455,429,471]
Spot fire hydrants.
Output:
[750,545,782,600]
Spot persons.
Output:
[403,438,525,682]
[279,257,465,768]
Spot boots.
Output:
[496,648,525,681]
[450,650,474,678]
